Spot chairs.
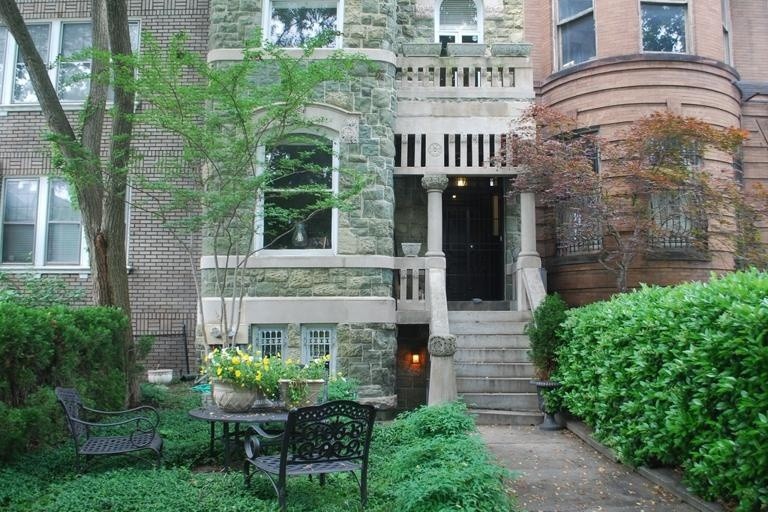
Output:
[241,397,379,512]
[53,384,165,472]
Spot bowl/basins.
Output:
[401,241,422,256]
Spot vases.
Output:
[279,377,326,413]
[208,377,258,413]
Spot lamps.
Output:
[411,347,420,365]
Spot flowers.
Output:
[198,341,284,402]
[279,353,334,410]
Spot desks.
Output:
[185,405,329,473]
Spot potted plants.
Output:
[520,290,571,432]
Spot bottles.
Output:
[291,223,309,247]
